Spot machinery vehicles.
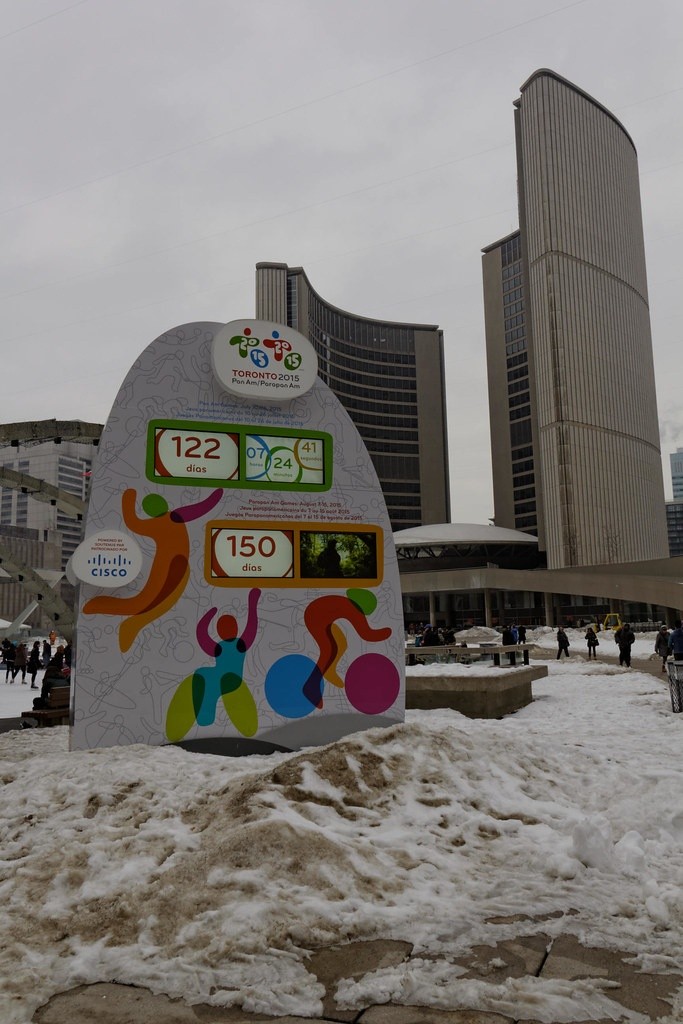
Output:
[591,613,623,635]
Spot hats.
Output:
[424,624,432,628]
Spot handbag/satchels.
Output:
[592,633,599,646]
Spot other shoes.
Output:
[31,684,39,689]
[12,680,14,683]
[10,680,13,683]
[5,679,8,683]
[22,681,27,684]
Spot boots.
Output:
[662,665,667,672]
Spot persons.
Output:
[614,623,635,669]
[502,622,526,645]
[557,626,570,660]
[585,627,597,661]
[654,625,673,673]
[409,622,457,647]
[668,619,683,661]
[1,637,73,690]
